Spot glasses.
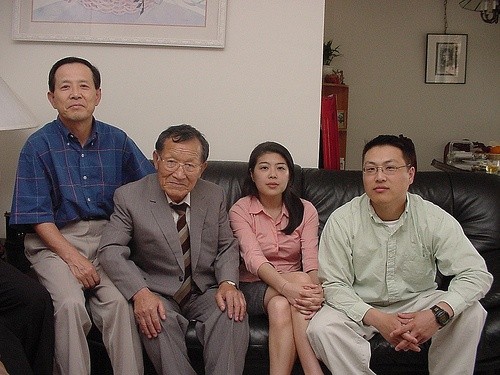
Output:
[157,151,204,172]
[363,164,411,175]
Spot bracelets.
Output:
[280,282,287,294]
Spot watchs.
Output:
[430,305,451,331]
[225,280,238,289]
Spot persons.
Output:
[0,57,157,375]
[230,141,326,375]
[98,123,249,375]
[307,135,494,375]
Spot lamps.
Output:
[458,0,500,24]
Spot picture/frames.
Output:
[424,33,468,84]
[12,0,227,49]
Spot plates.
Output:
[462,160,491,164]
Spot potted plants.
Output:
[323,40,344,79]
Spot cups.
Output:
[485,159,499,174]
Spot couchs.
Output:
[127,160,500,375]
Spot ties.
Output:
[168,202,193,307]
